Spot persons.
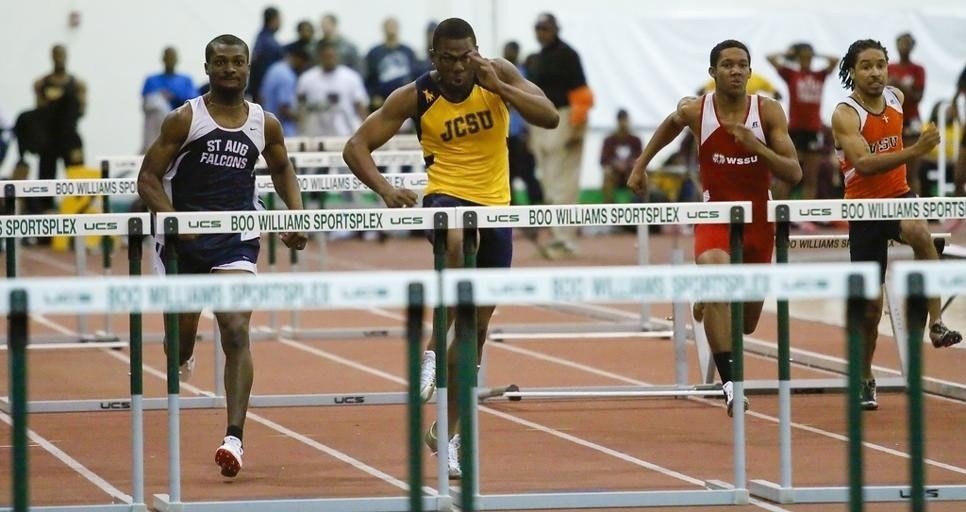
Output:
[496,14,592,260]
[340,15,562,483]
[141,47,193,116]
[361,18,439,239]
[886,33,966,198]
[134,32,309,480]
[696,39,846,233]
[623,39,806,421]
[600,109,649,207]
[828,38,964,412]
[247,7,381,242]
[33,44,86,243]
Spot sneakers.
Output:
[214,435,245,478]
[179,355,196,383]
[859,373,879,409]
[724,380,749,419]
[424,419,463,478]
[419,350,439,403]
[929,323,962,348]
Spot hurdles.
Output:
[0,175,229,416]
[891,258,966,511]
[742,197,966,504]
[151,205,457,511]
[1,212,152,511]
[1,268,440,512]
[439,260,881,511]
[1,136,966,404]
[448,196,754,509]
[197,173,442,407]
